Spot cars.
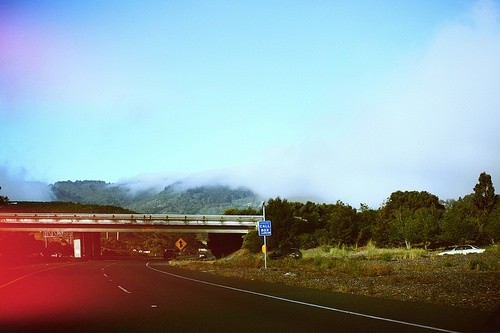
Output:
[436,245,485,255]
[267,247,303,261]
